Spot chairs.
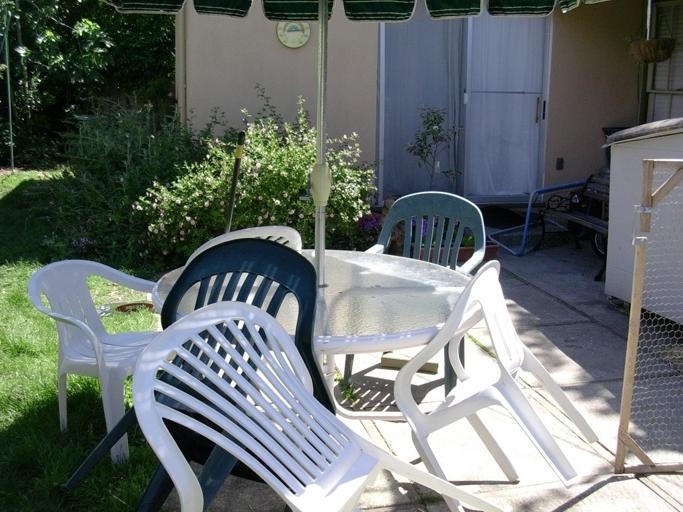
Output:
[395,260,598,512]
[58,238,336,512]
[186,226,303,252]
[129,300,498,512]
[342,189,488,398]
[29,259,163,466]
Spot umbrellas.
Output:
[105,1,611,288]
